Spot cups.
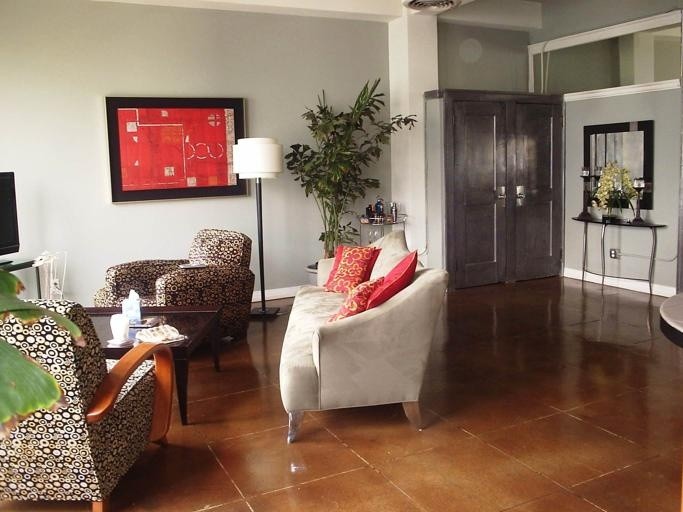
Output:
[122,300,141,326]
[109,314,128,341]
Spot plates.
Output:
[178,264,206,269]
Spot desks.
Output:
[572,216,668,304]
[659,291,683,348]
[1,258,42,300]
[359,213,408,246]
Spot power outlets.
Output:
[610,248,620,259]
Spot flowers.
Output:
[592,161,638,216]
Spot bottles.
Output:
[366,204,383,223]
[375,200,382,213]
[391,202,398,222]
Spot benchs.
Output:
[279,228,449,443]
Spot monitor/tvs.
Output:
[0,171,20,266]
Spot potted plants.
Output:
[285,75,419,285]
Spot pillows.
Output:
[328,276,384,322]
[323,242,382,294]
[366,248,417,310]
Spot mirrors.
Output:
[583,119,654,210]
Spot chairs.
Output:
[94,228,255,343]
[0,298,175,512]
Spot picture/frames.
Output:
[105,96,249,204]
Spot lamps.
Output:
[631,178,645,225]
[577,166,593,220]
[232,137,284,319]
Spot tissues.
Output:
[121,289,141,319]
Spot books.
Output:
[128,316,160,328]
